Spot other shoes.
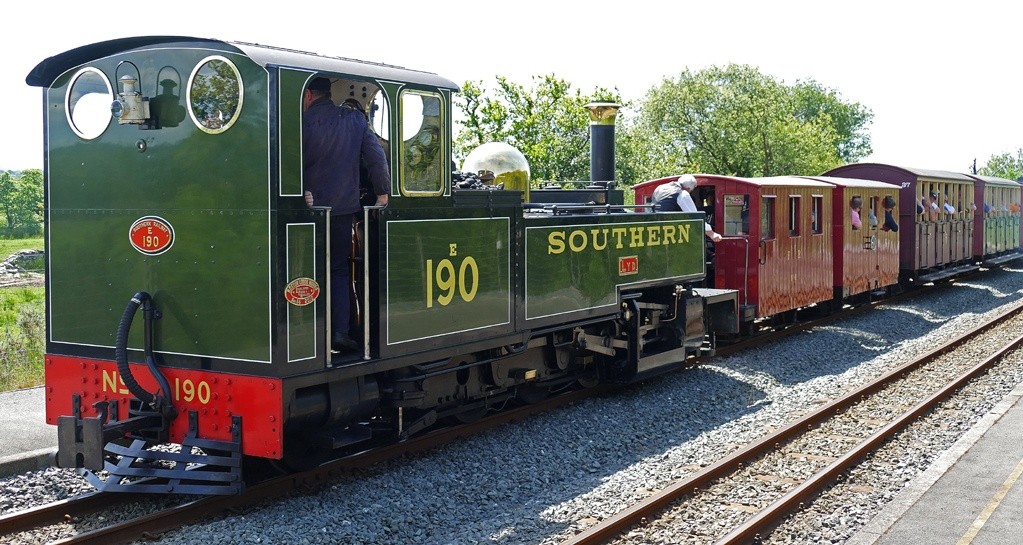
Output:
[331,343,360,358]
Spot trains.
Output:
[23,34,1023,504]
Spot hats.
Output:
[930,192,937,200]
[883,197,895,208]
[309,77,331,90]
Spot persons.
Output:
[917,191,1020,215]
[885,198,898,232]
[850,198,863,230]
[870,209,878,226]
[701,191,715,256]
[651,174,722,243]
[302,77,391,363]
